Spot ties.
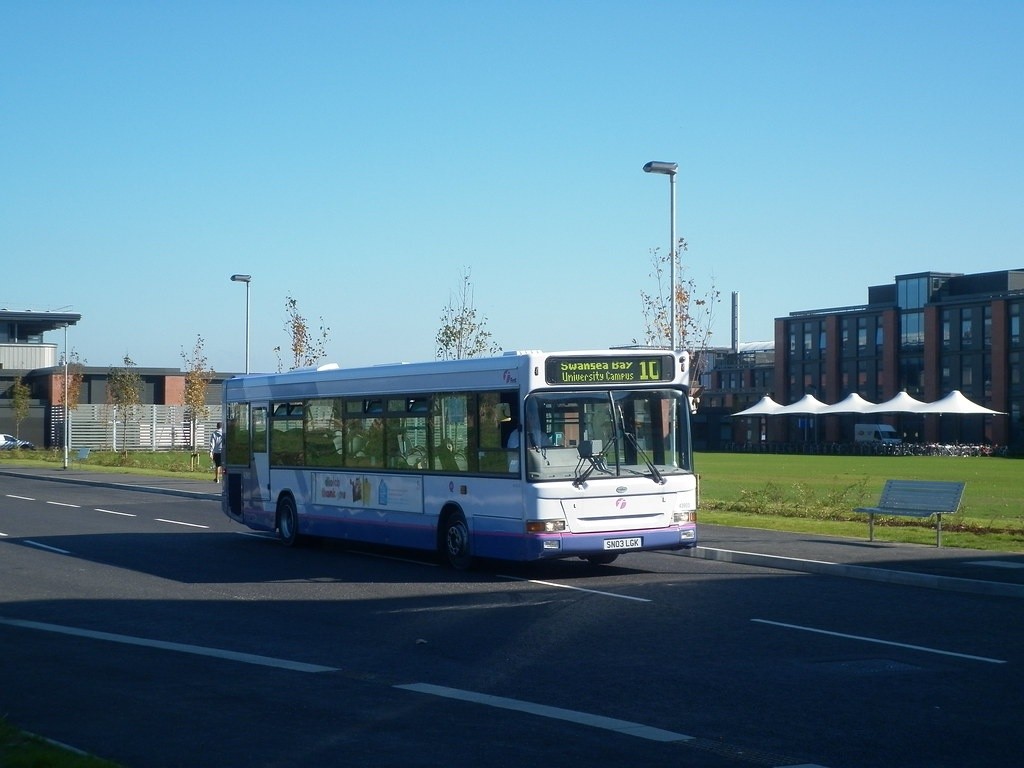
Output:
[528,432,537,447]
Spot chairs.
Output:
[236,428,468,474]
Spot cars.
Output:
[0,433,35,452]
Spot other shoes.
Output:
[213,479,219,483]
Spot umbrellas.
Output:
[866,391,928,444]
[917,390,1007,445]
[731,397,784,441]
[771,394,828,442]
[816,393,876,443]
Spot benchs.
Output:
[71,448,90,469]
[853,479,966,548]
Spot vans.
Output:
[854,423,903,447]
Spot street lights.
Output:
[642,161,680,350]
[230,273,251,375]
[53,322,69,469]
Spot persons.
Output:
[507,411,554,472]
[209,422,222,483]
[442,438,454,452]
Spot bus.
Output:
[221,346,701,575]
